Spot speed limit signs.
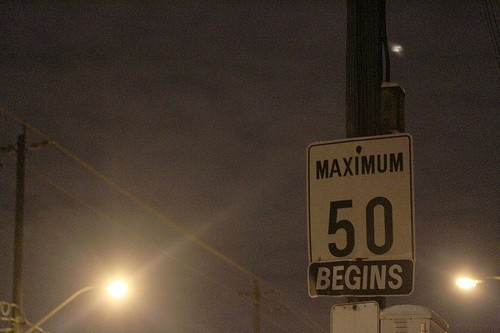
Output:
[304,132,416,301]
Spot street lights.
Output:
[19,275,128,333]
